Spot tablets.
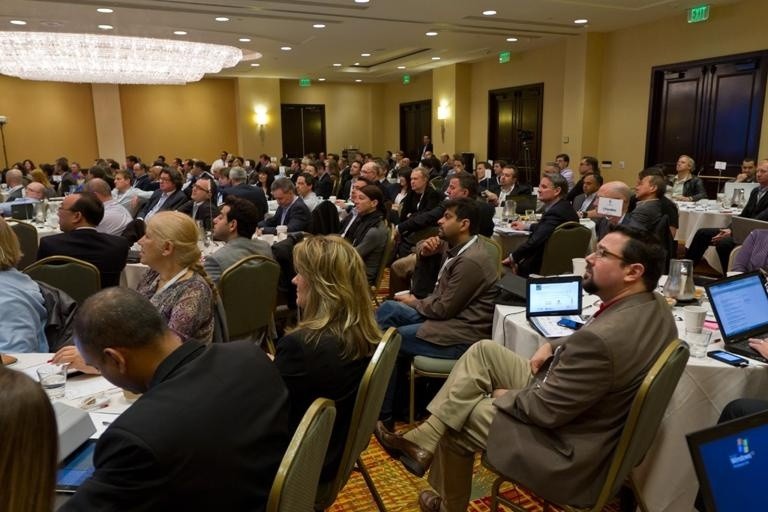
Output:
[52,438,102,494]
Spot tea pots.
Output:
[664,260,697,305]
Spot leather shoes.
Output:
[375,420,433,478]
[419,489,448,512]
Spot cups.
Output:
[38,364,65,403]
[258,225,288,242]
[123,386,142,405]
[494,199,537,227]
[686,328,714,359]
[32,201,62,231]
[684,306,708,334]
[329,196,336,203]
[194,219,214,256]
[571,258,588,277]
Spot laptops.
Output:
[525,276,585,339]
[724,181,761,205]
[684,408,767,512]
[702,269,768,363]
[731,216,768,245]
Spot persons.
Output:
[55,287,295,511]
[0,368,58,511]
[269,234,382,483]
[0,134,767,433]
[376,225,679,511]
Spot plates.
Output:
[678,201,742,216]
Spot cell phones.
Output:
[707,350,749,367]
[557,318,583,330]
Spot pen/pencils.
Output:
[708,338,721,345]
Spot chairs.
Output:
[3,217,103,302]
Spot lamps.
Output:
[0,30,245,87]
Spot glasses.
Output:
[594,244,625,266]
[158,177,171,184]
[191,184,209,193]
